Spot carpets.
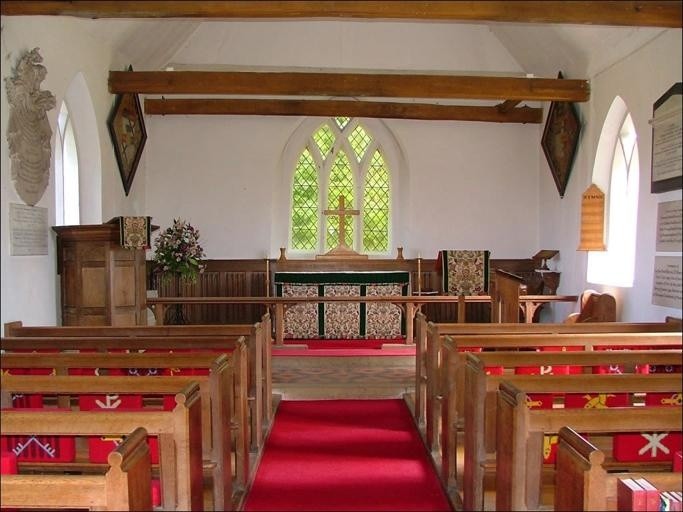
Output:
[234,396,456,512]
[273,349,413,355]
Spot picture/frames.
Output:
[539,70,587,198]
[107,65,149,196]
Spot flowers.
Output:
[152,217,209,286]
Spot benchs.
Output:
[6,320,262,452]
[428,321,679,451]
[0,336,250,488]
[0,352,234,510]
[558,424,683,511]
[494,374,683,511]
[0,426,152,512]
[428,318,683,486]
[440,334,683,510]
[2,379,206,512]
[415,313,683,427]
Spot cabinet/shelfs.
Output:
[50,222,160,327]
